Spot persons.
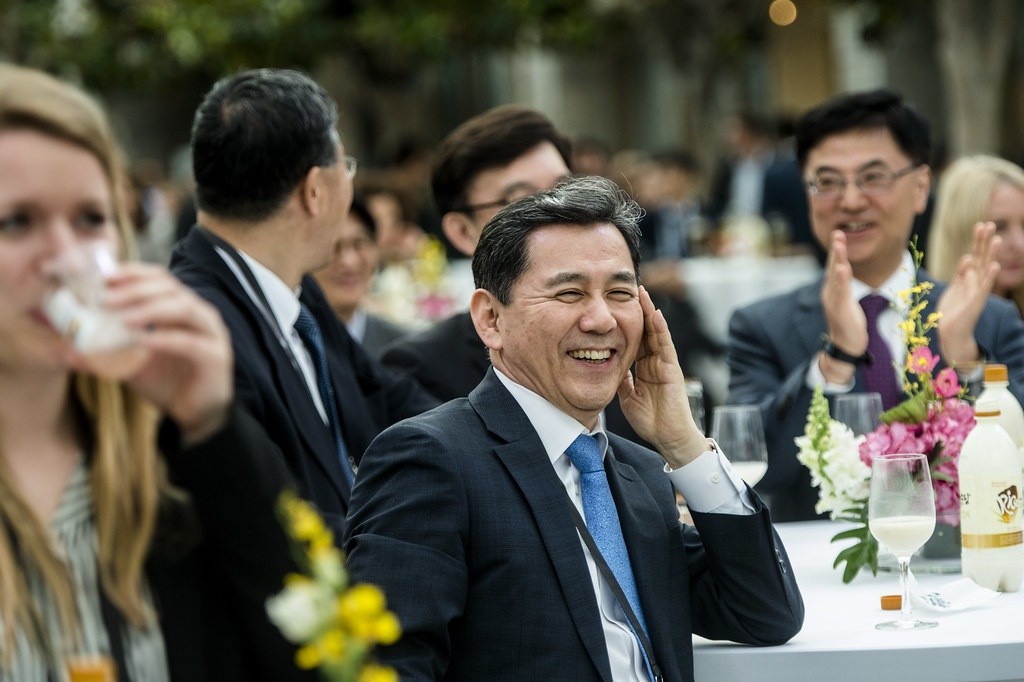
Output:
[345,174,806,682]
[0,60,327,682]
[143,67,452,682]
[318,129,460,360]
[366,103,726,439]
[721,87,1024,521]
[911,136,1024,320]
[123,138,196,279]
[563,108,827,271]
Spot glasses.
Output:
[804,162,922,199]
[453,173,570,212]
[314,157,357,177]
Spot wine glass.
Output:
[869,454,939,635]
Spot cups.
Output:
[714,405,767,491]
[835,394,885,438]
[22,240,155,382]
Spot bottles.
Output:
[671,378,706,513]
[974,365,1021,456]
[959,407,1022,595]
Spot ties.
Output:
[859,296,899,411]
[292,302,355,487]
[565,434,665,682]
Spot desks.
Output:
[688,519,1024,682]
[680,254,828,347]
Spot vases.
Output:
[918,522,962,560]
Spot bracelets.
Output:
[814,334,876,371]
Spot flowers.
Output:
[263,486,402,682]
[411,236,454,322]
[793,234,976,584]
